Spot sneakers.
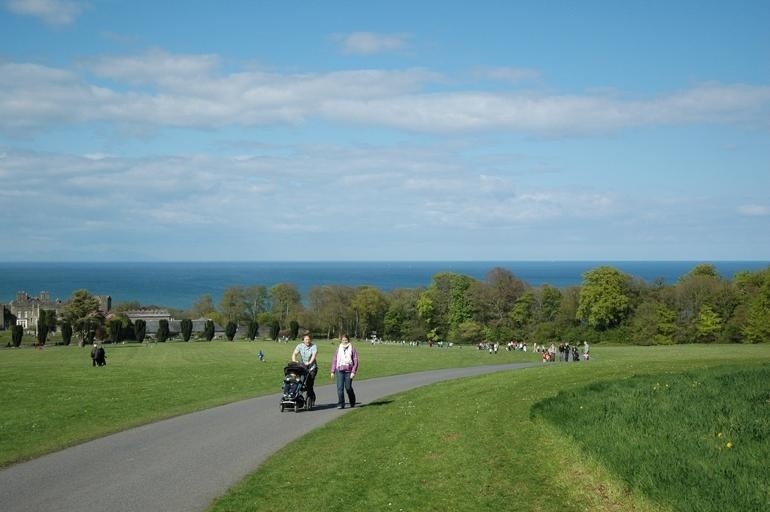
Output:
[337,406,344,409]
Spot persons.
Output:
[284,372,300,398]
[291,335,318,406]
[90,344,106,366]
[403,340,588,362]
[257,350,263,361]
[331,333,358,409]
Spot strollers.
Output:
[278,362,313,412]
